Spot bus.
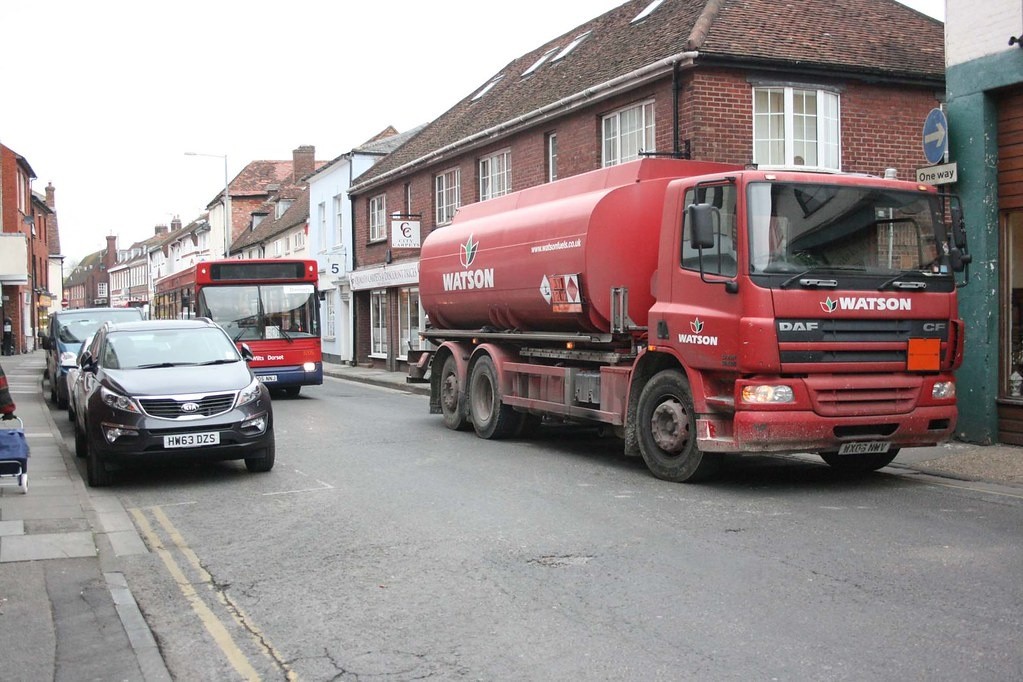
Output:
[113,301,148,308]
[153,259,326,396]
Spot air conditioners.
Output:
[94,299,107,305]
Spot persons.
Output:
[284,316,302,332]
[3,311,16,356]
[137,298,144,308]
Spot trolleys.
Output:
[0,414,29,493]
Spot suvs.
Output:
[71,317,275,487]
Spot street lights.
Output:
[184,152,229,260]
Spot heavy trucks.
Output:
[407,158,973,482]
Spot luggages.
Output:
[0,414,28,494]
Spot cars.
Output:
[60,336,171,422]
[38,307,146,410]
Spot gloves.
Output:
[2,412,13,420]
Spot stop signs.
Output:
[61,299,68,306]
